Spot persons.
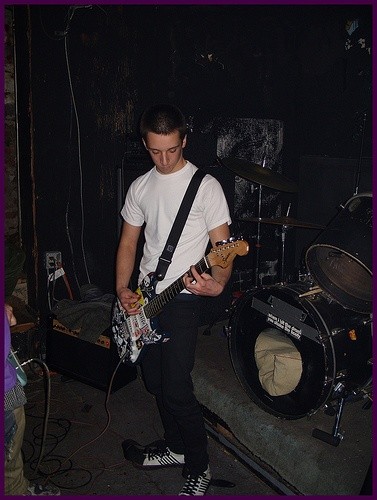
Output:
[4,303,61,495]
[116,103,234,496]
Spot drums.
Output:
[304,190,377,315]
[225,271,377,422]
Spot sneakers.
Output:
[133,446,185,469]
[22,483,60,496]
[178,464,212,496]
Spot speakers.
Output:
[117,151,234,291]
[45,312,139,394]
[297,152,372,282]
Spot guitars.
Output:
[109,233,250,367]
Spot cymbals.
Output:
[219,156,302,194]
[231,216,341,233]
